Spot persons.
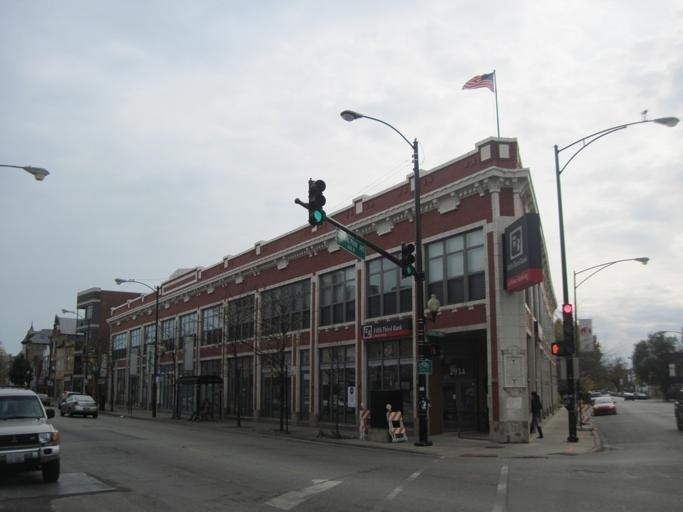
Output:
[530,391,543,438]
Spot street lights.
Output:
[340,110,441,446]
[554,116,681,443]
[114,278,167,417]
[0,164,50,181]
[572,256,650,429]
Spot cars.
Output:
[674,389,683,430]
[624,392,648,401]
[0,388,98,482]
[593,397,617,415]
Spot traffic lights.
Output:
[551,303,574,355]
[402,243,416,278]
[308,178,326,226]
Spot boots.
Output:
[537,426,544,438]
[530,423,534,433]
